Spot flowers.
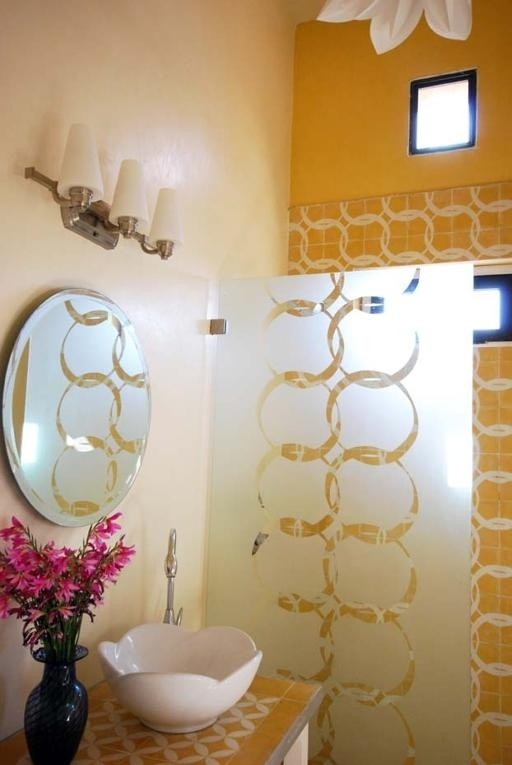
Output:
[0,511,140,639]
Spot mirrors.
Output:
[0,285,155,529]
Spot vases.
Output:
[23,645,89,765]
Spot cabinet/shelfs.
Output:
[279,722,310,765]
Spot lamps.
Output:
[23,120,187,262]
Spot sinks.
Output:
[98,621,263,734]
[162,526,184,627]
